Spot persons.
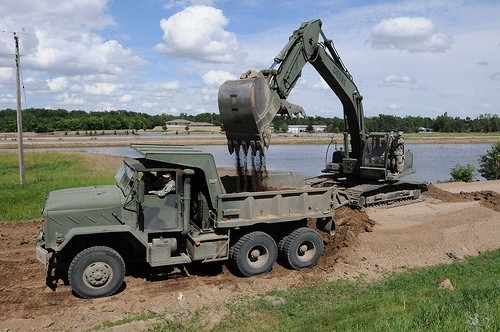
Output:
[148,173,176,197]
[140,172,157,181]
[391,131,404,175]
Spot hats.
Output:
[162,173,173,178]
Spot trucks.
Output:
[36,144,335,298]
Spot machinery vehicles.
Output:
[218,19,428,212]
